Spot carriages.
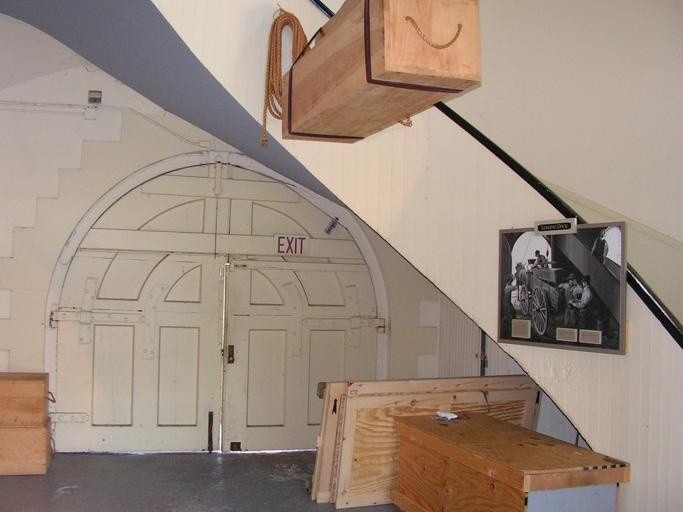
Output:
[515,263,565,335]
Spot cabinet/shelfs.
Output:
[389,410,631,512]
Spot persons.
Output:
[503,247,593,330]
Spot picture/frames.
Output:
[496,221,625,356]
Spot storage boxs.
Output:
[1,372,52,474]
[280,0,482,144]
